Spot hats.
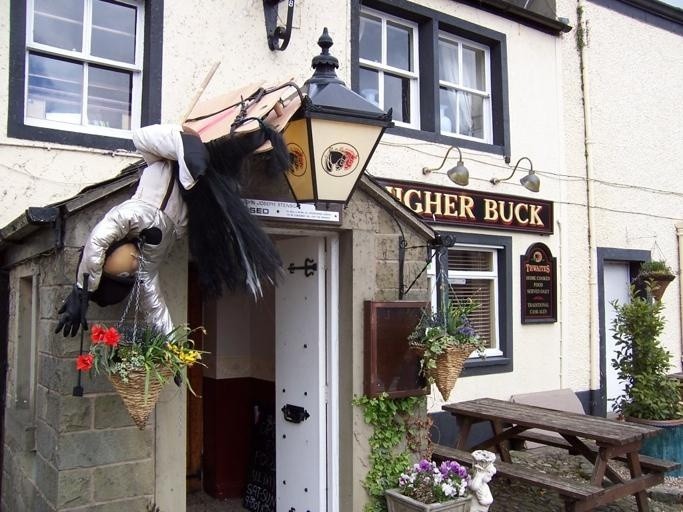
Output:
[89,271,135,307]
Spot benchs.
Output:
[514,420,683,478]
[424,439,604,512]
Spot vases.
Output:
[382,485,467,511]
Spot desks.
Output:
[437,393,650,512]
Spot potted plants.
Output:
[608,258,682,481]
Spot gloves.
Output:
[54,285,89,338]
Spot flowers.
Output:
[394,456,471,504]
[71,316,209,407]
[405,283,498,386]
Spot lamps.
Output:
[271,24,398,214]
[492,155,541,193]
[422,143,473,187]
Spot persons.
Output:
[466,448,496,512]
